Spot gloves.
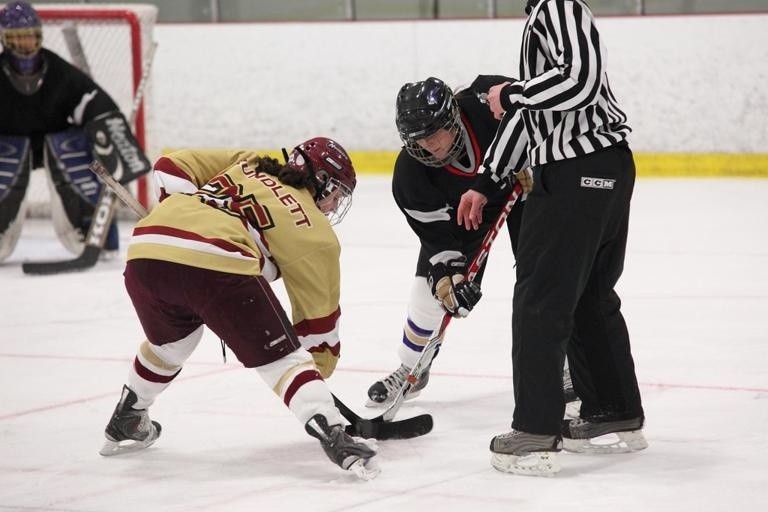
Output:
[425,257,478,319]
[509,163,536,202]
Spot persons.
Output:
[457,0,650,477]
[367,74,521,408]
[0,0,119,266]
[98,137,381,481]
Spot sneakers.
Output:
[562,416,645,440]
[104,385,161,444]
[489,428,563,454]
[368,363,431,403]
[563,370,579,403]
[305,414,380,469]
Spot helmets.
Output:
[281,137,356,227]
[0,2,48,95]
[395,76,466,167]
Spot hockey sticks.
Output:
[20,40,160,277]
[87,157,434,441]
[344,178,523,438]
